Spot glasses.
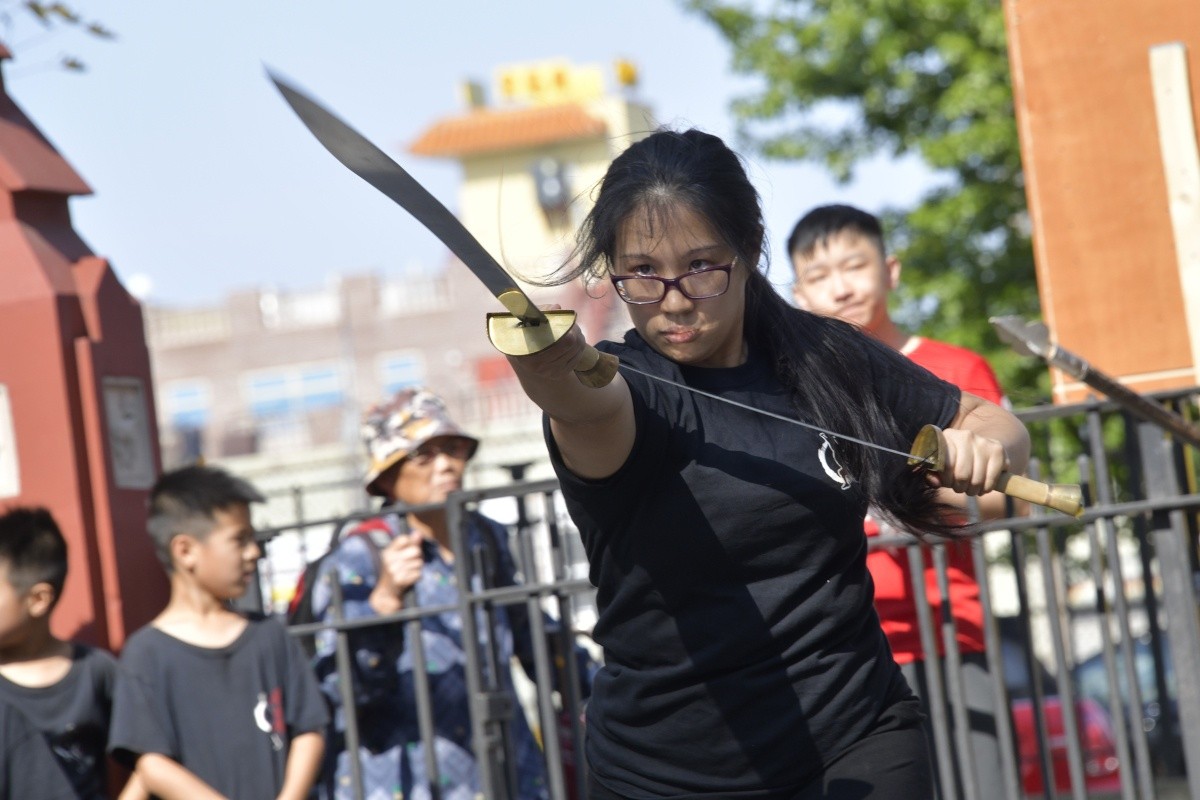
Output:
[605,254,744,306]
[410,439,470,462]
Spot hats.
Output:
[356,386,482,498]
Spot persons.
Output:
[108,467,330,800]
[288,385,606,800]
[785,205,1031,800]
[0,504,138,800]
[505,130,1030,800]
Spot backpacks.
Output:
[289,518,406,743]
